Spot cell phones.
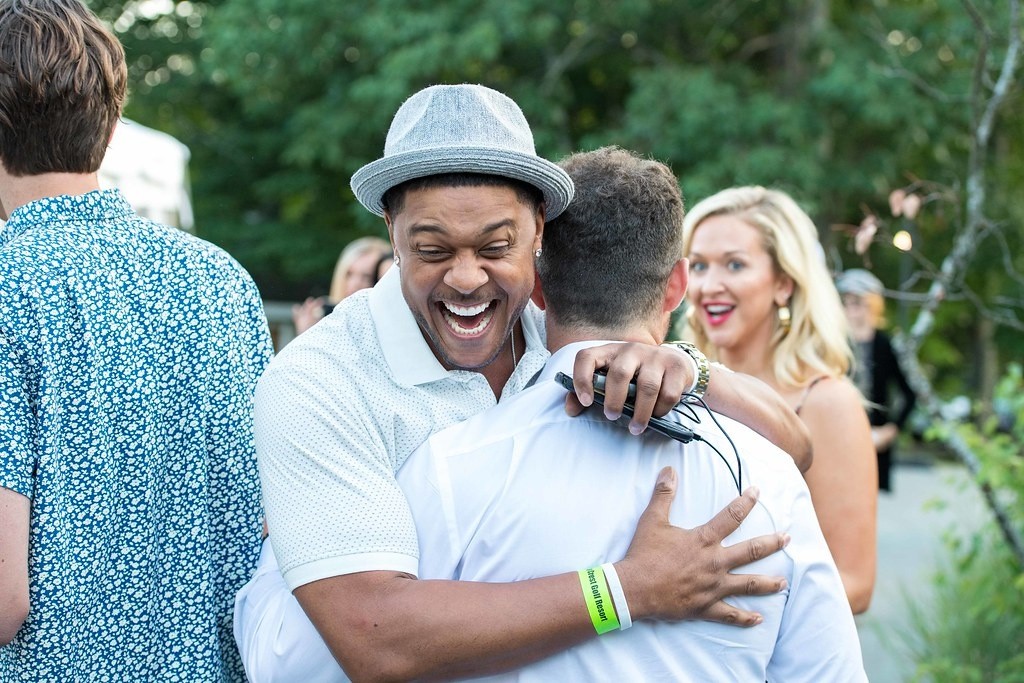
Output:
[554,371,694,444]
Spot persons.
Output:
[253,83,813,682]
[234,145,868,683]
[836,269,916,488]
[0,1,277,683]
[326,234,396,303]
[684,183,879,614]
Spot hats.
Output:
[349,83,574,222]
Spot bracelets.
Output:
[663,340,710,403]
[580,562,633,635]
